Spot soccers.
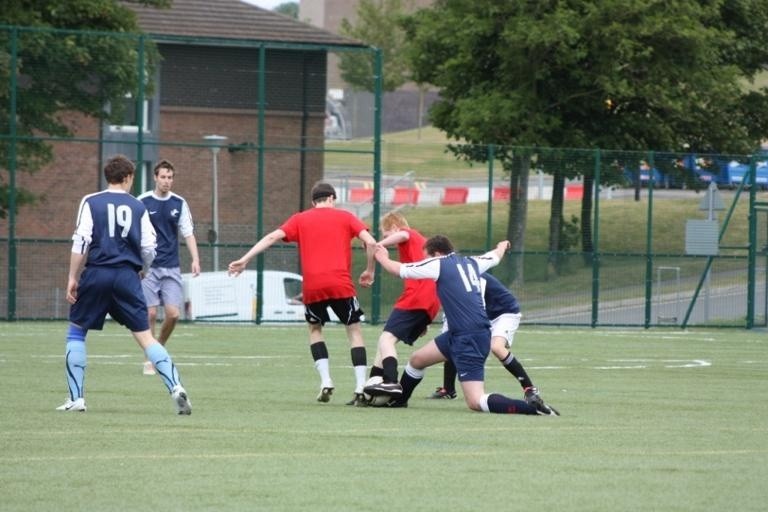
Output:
[364,376,391,406]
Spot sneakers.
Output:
[427,387,457,399]
[355,381,408,408]
[524,387,560,417]
[318,380,334,402]
[143,360,155,374]
[55,397,86,411]
[172,385,192,415]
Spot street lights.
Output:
[204,135,226,271]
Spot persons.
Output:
[373,233,562,416]
[359,209,441,396]
[425,270,545,409]
[227,181,379,406]
[134,159,203,377]
[52,153,195,416]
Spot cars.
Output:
[610,149,768,190]
